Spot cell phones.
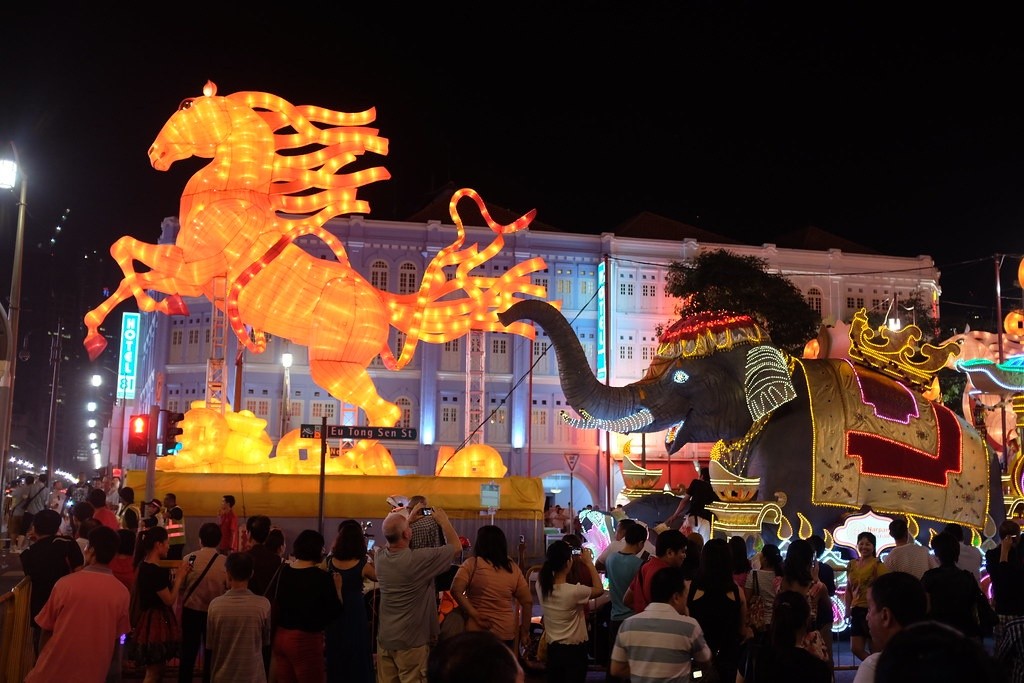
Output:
[421,507,434,516]
[367,539,375,551]
[571,548,581,555]
[188,555,196,566]
[520,535,524,544]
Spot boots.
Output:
[3,541,9,548]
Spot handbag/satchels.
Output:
[261,594,280,621]
[749,570,765,626]
[22,502,29,510]
[440,610,467,639]
[535,630,546,661]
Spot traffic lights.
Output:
[160,409,184,460]
[128,411,147,458]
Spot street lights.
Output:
[87,402,115,475]
[279,352,295,442]
[91,365,128,488]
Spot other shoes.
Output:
[9,547,22,553]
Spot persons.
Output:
[204,515,379,683]
[544,502,617,549]
[375,496,533,683]
[0,473,239,683]
[535,519,836,683]
[610,504,622,511]
[666,468,720,547]
[844,519,1024,683]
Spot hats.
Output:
[146,499,161,507]
